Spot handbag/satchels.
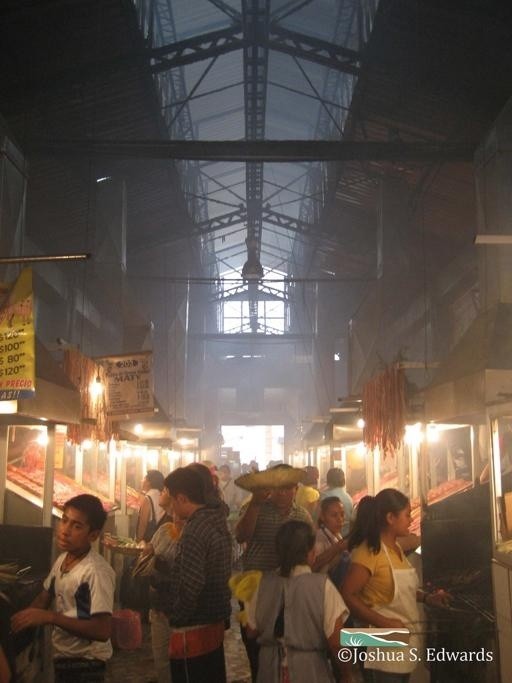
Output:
[137,516,156,542]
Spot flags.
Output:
[1,266,39,402]
[104,351,156,421]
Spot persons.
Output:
[133,458,456,682]
[7,492,117,683]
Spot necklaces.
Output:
[60,547,89,578]
